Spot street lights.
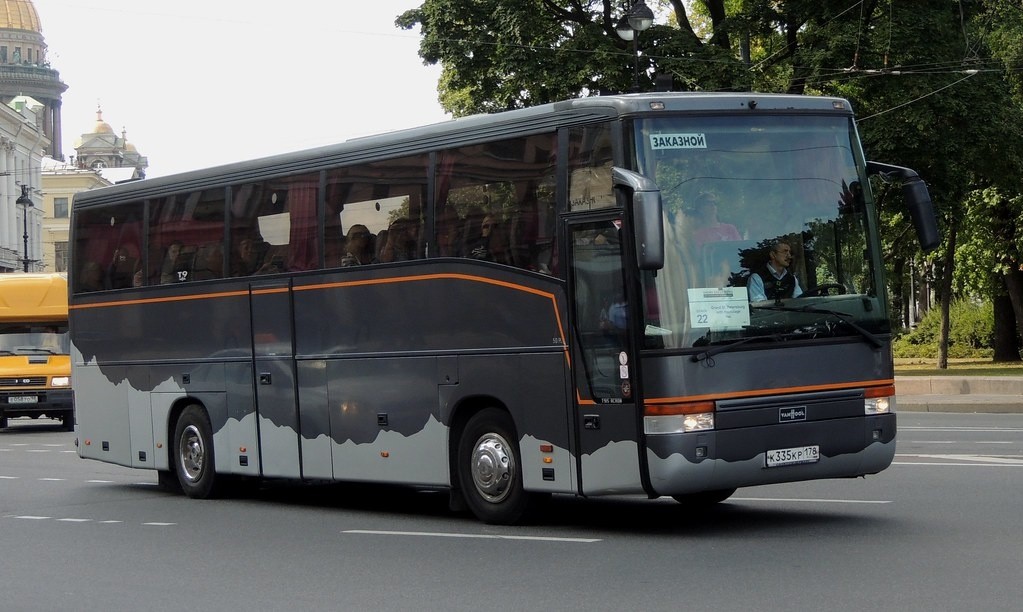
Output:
[16,186,34,273]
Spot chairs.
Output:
[263,245,287,263]
[191,244,223,281]
[104,258,135,290]
[374,230,387,263]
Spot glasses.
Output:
[705,200,718,205]
[483,224,500,228]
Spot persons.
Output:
[746,238,803,303]
[600,288,627,347]
[104,214,553,287]
[690,192,743,247]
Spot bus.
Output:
[67,93,946,525]
[0,273,74,432]
